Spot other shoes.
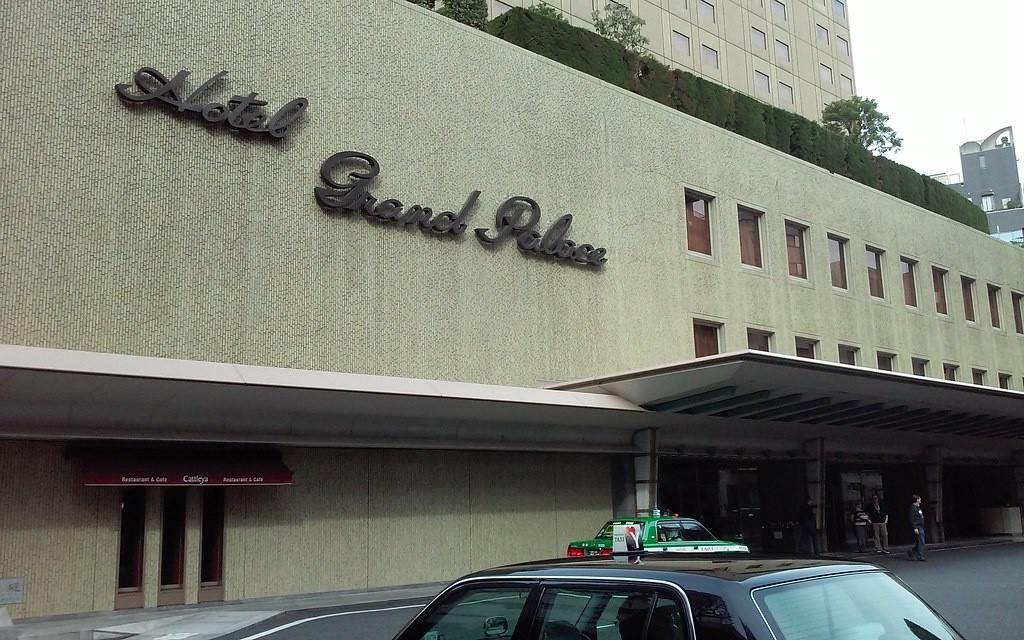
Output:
[877,550,882,553]
[883,549,890,554]
[908,550,915,560]
[918,558,924,561]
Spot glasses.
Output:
[614,619,622,627]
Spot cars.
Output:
[392,555,970,640]
[564,510,751,556]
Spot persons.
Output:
[854,500,872,553]
[801,498,826,559]
[907,494,928,563]
[867,494,891,554]
[613,595,650,640]
[697,512,705,526]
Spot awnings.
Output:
[69,445,292,486]
